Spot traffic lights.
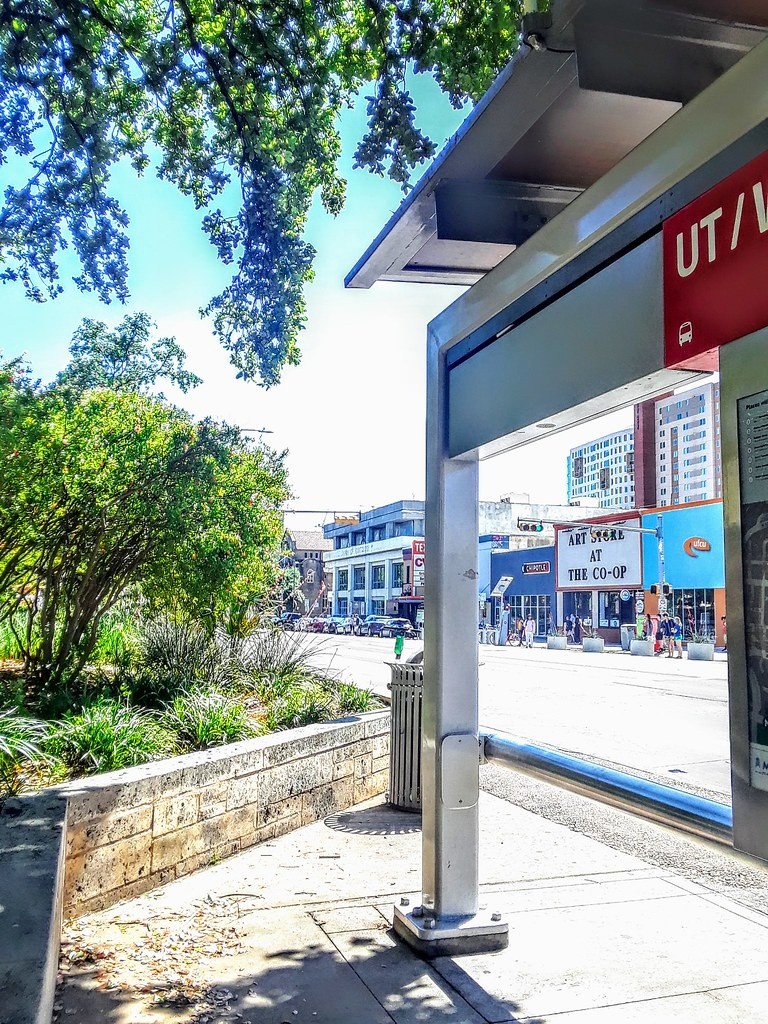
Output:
[520,523,545,534]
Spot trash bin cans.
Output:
[383,651,425,813]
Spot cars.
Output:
[258,611,417,638]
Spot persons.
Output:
[661,611,676,658]
[522,614,536,648]
[721,616,727,651]
[354,614,360,634]
[350,615,355,635]
[573,615,581,644]
[655,613,665,652]
[516,614,524,646]
[565,616,576,644]
[644,613,654,639]
[673,616,684,659]
